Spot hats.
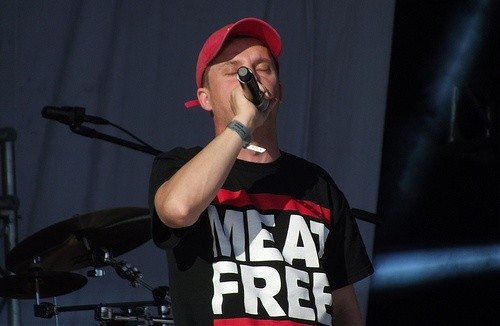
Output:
[185,18,283,108]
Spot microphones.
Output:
[237,65,269,113]
[40,105,111,125]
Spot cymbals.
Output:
[1,270,88,299]
[0,208,153,270]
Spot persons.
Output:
[150,16,374,326]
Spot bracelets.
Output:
[228,120,252,148]
